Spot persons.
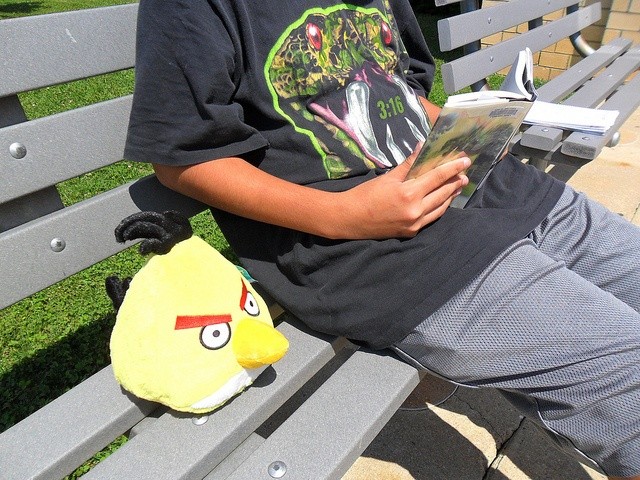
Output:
[122,0,637,476]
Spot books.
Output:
[402,47,538,212]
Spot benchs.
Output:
[435,0,640,173]
[0,4,491,480]
